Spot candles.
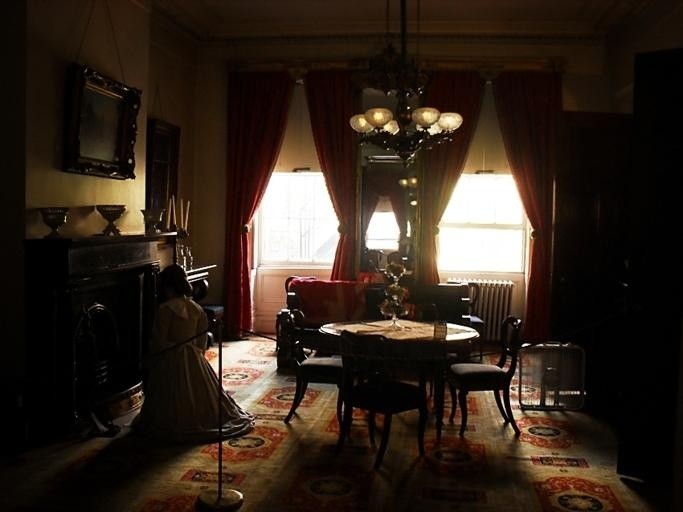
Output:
[165,195,190,229]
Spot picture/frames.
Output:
[57,56,142,181]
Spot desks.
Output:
[318,320,481,443]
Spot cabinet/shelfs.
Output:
[24,232,177,428]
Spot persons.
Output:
[123,264,251,445]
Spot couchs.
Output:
[275,275,468,374]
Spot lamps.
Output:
[348,1,464,168]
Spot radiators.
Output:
[470,282,514,342]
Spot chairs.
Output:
[281,309,427,467]
[468,282,484,360]
[448,316,522,438]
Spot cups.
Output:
[433,320,448,339]
